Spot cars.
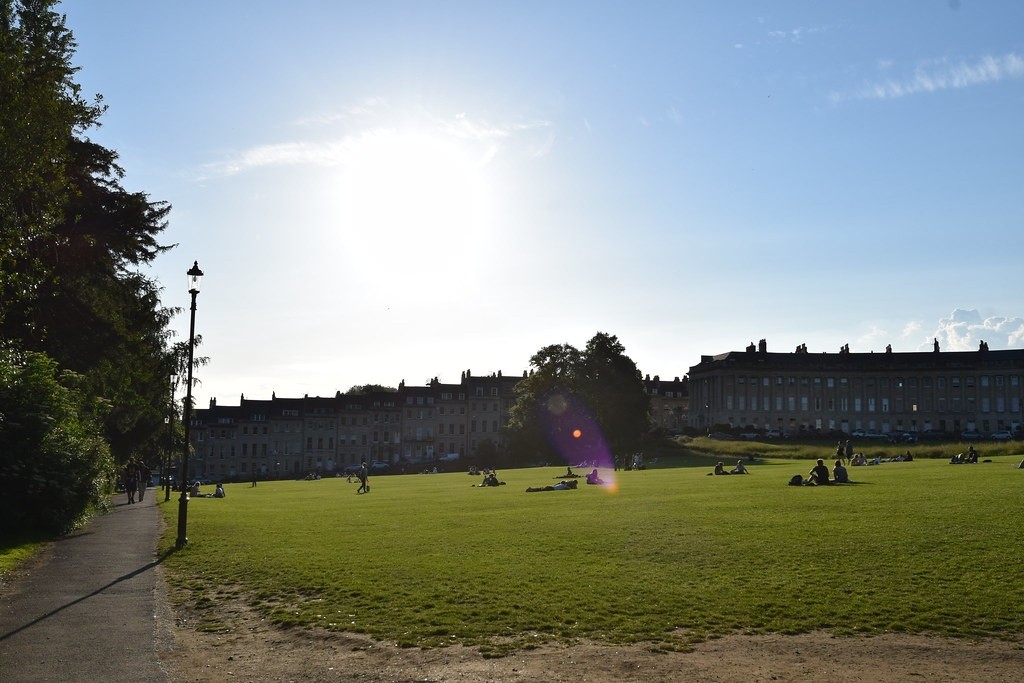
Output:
[852,430,866,438]
[741,432,760,438]
[708,432,733,439]
[372,462,389,468]
[344,463,363,471]
[961,431,984,439]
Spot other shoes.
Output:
[139,499,143,502]
[128,498,134,505]
[803,481,808,484]
[526,487,531,492]
[357,490,359,493]
[364,491,367,493]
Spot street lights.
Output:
[177,259,203,545]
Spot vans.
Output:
[991,430,1013,440]
[439,453,458,461]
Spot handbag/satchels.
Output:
[357,471,363,479]
[366,485,370,491]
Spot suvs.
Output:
[864,430,887,439]
[917,428,945,439]
[766,429,793,439]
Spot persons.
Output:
[830,460,848,483]
[715,460,749,475]
[468,464,481,475]
[525,480,578,492]
[172,477,226,499]
[400,466,446,475]
[302,470,361,483]
[614,452,660,471]
[115,457,153,505]
[554,459,605,485]
[837,440,913,466]
[253,469,257,487]
[357,462,369,493]
[951,446,977,464]
[749,455,753,460]
[481,465,501,487]
[807,459,829,485]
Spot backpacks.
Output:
[788,475,802,485]
